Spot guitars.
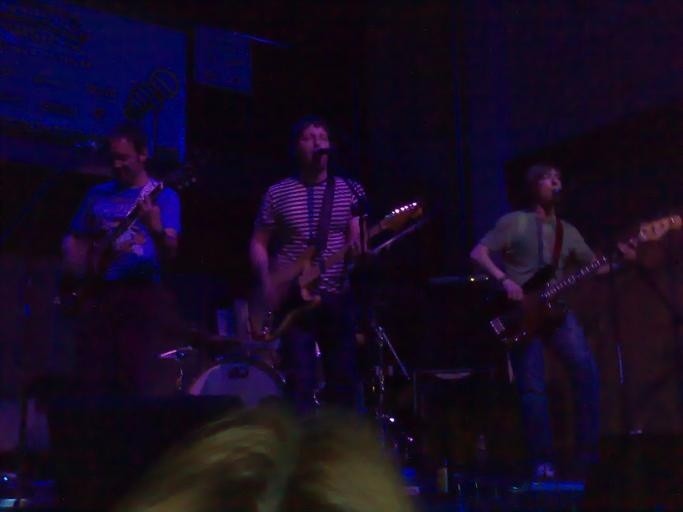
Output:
[484,213,683,344]
[250,203,423,340]
[62,178,167,313]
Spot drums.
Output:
[183,359,297,409]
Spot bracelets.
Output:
[497,276,511,285]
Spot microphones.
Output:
[312,143,330,157]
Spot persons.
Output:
[248,116,367,412]
[468,158,639,477]
[111,399,413,511]
[61,123,183,392]
